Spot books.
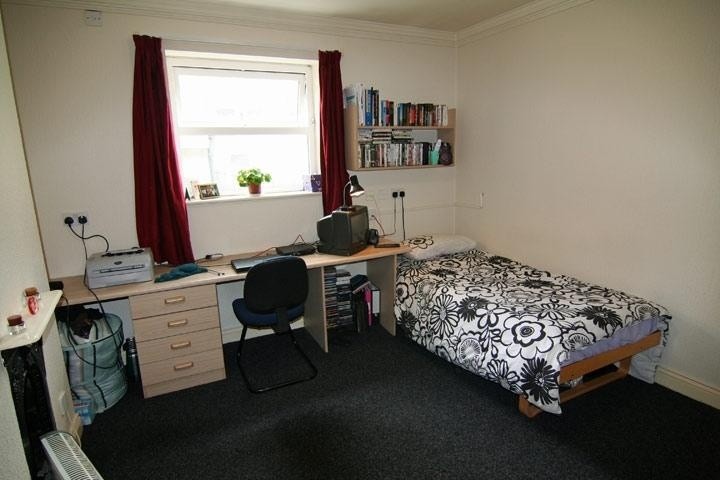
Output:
[343,82,448,168]
[324,267,354,330]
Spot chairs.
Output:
[232,256,318,394]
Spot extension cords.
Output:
[49,282,63,290]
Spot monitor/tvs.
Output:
[317,206,369,256]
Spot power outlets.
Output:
[390,187,405,200]
[368,208,375,220]
[60,209,91,227]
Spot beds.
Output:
[393,249,672,419]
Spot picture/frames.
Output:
[197,183,221,200]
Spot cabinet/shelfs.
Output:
[343,101,457,172]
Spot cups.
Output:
[430,151,440,164]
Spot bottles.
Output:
[124,336,141,383]
[6,315,31,343]
[24,287,42,316]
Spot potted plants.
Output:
[236,169,272,194]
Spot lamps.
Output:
[339,175,365,211]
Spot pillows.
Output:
[400,233,477,261]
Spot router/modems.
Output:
[276,243,315,256]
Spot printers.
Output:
[86,247,155,289]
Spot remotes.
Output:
[348,205,356,211]
[341,205,348,211]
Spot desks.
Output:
[47,236,412,400]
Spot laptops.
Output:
[231,255,291,272]
